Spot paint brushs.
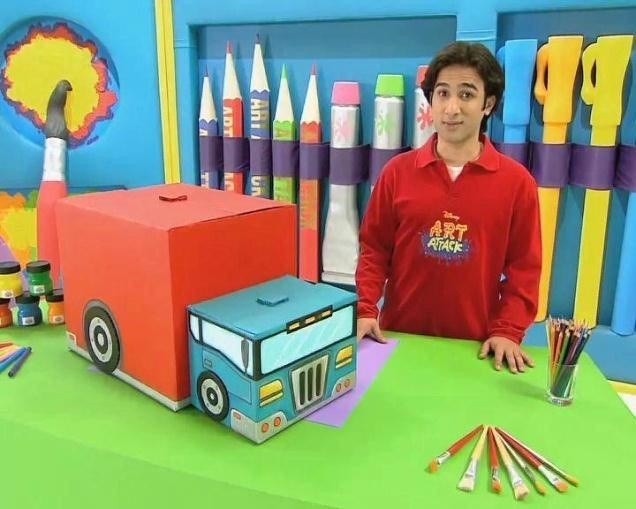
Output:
[430,423,579,500]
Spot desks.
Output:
[0,313,636,509]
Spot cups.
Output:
[546,361,577,406]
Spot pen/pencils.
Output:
[199,34,322,282]
[0,340,31,377]
[525,36,585,325]
[579,36,634,330]
[610,151,636,336]
[496,38,538,174]
[545,314,591,398]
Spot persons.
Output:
[357,42,543,373]
